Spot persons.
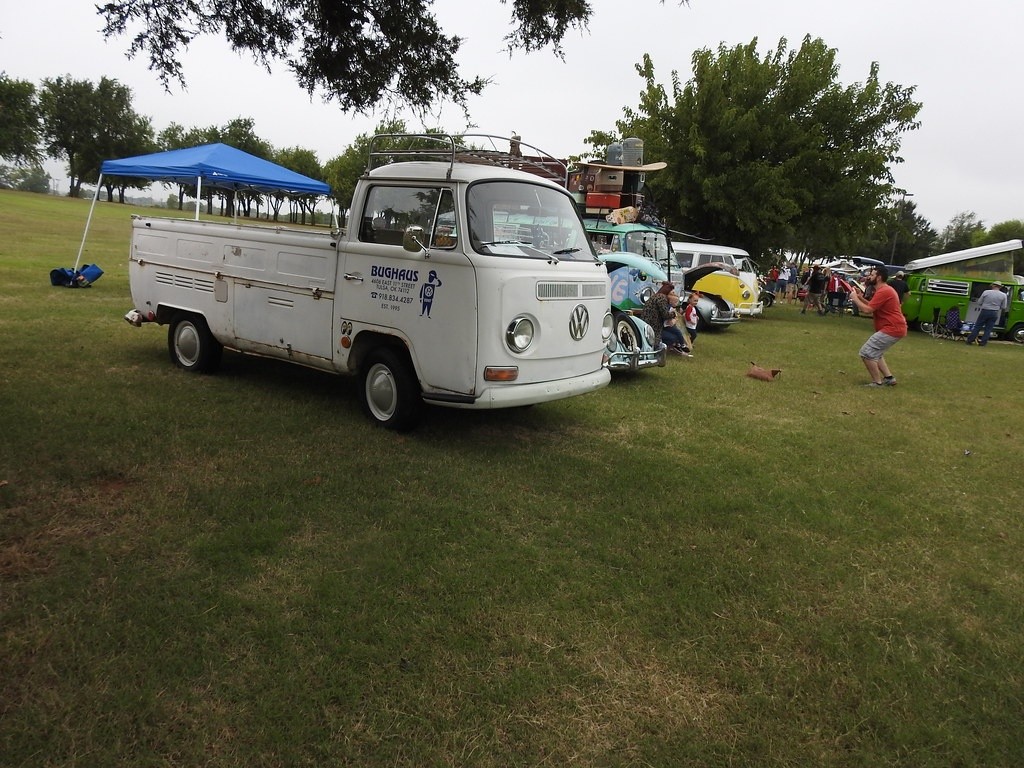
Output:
[849,266,907,385]
[770,261,872,316]
[887,271,911,304]
[966,281,1007,346]
[640,281,698,357]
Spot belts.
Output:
[982,309,998,311]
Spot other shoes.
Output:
[780,301,783,304]
[669,344,691,355]
[801,310,806,314]
[817,310,825,317]
[774,301,776,304]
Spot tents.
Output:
[74,142,340,273]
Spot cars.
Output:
[839,255,904,281]
[685,261,741,331]
[598,252,668,372]
[757,279,776,308]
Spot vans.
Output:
[900,274,1024,343]
[670,240,764,334]
[452,210,683,316]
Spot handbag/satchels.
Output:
[803,284,810,292]
[747,361,781,382]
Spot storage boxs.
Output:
[570,165,647,225]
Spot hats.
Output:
[896,271,904,277]
[990,281,1004,287]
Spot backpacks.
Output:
[812,272,825,290]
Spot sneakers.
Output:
[865,381,884,388]
[882,375,897,385]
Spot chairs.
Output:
[823,291,848,317]
[925,305,979,346]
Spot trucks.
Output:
[123,132,614,428]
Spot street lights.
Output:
[890,193,914,265]
